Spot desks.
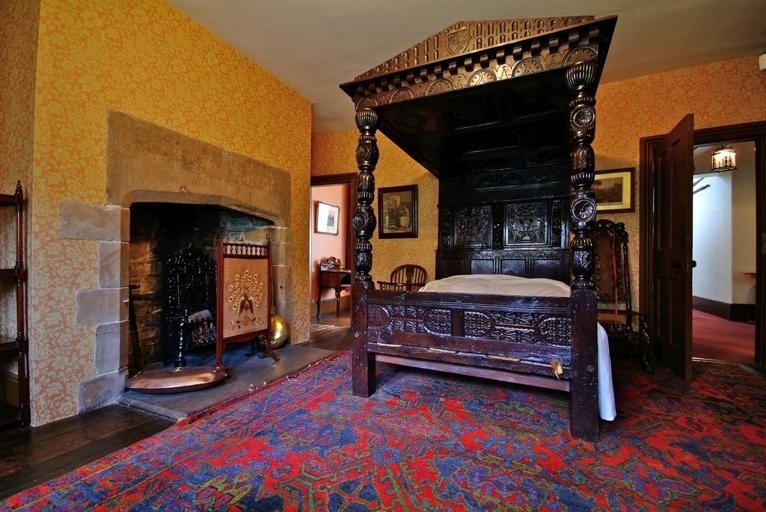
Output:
[316,270,351,320]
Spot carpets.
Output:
[1,347,766,512]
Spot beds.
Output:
[337,16,615,443]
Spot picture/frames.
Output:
[589,167,636,214]
[314,201,339,235]
[378,184,418,238]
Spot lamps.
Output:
[711,145,736,172]
